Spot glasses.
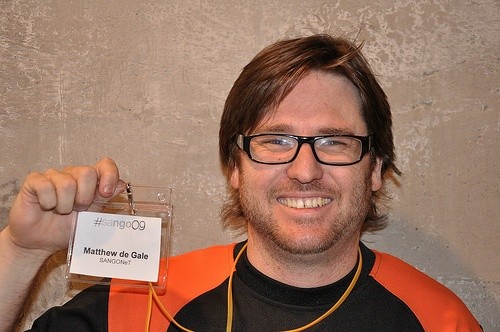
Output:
[235,132,375,167]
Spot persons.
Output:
[0,34,484,332]
